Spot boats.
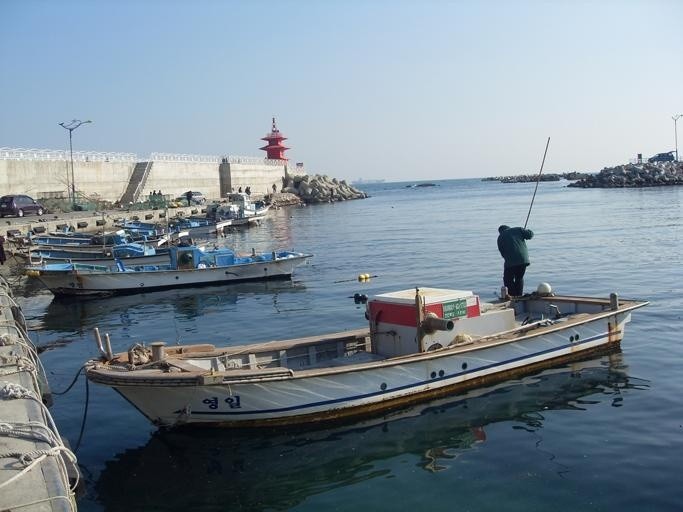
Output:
[82,285,650,441]
[174,190,273,233]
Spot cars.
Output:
[1,195,44,216]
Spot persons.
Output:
[272,184,276,193]
[149,190,162,195]
[238,187,251,195]
[187,191,192,206]
[497,225,533,298]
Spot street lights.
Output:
[59,120,92,209]
[672,115,683,162]
